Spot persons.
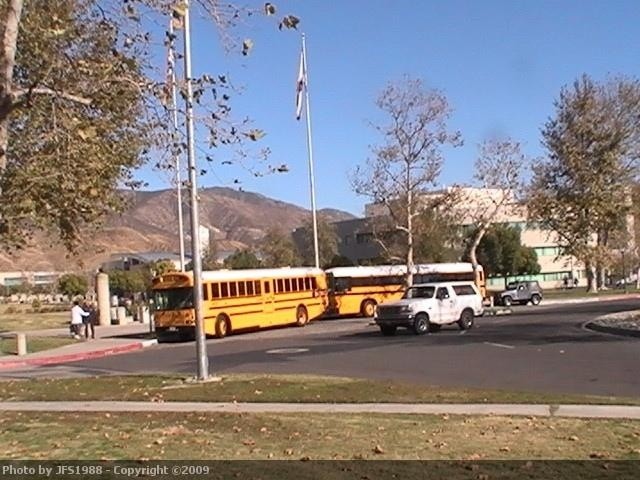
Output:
[70,301,95,342]
[561,274,579,288]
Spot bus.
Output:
[319,262,488,319]
[149,267,330,343]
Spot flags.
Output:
[295,46,307,121]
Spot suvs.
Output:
[375,280,485,335]
[488,280,543,307]
